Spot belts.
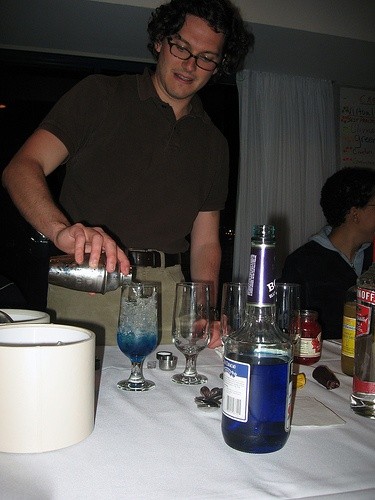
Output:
[82,251,181,268]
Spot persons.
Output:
[280,166,375,341]
[0,0,256,349]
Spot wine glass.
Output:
[117,280,160,392]
[218,282,247,379]
[171,281,212,386]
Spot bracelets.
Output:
[195,306,220,321]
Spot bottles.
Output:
[222,223,293,453]
[48,253,133,296]
[350,240,375,420]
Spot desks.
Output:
[0,341,374,500]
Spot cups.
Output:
[0,308,51,326]
[0,323,97,453]
[275,282,301,347]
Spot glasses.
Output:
[165,35,223,72]
